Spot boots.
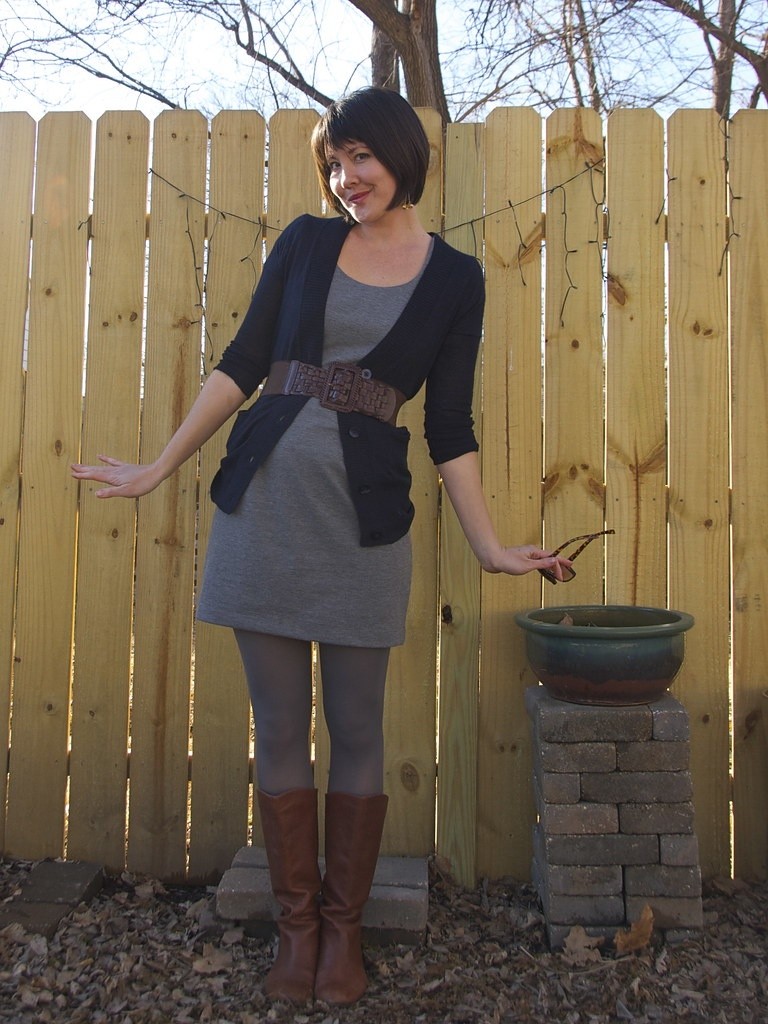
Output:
[315,794,389,1006]
[257,788,322,1004]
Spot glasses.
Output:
[537,530,615,584]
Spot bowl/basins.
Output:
[513,605,695,707]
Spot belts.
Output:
[260,361,405,426]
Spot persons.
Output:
[72,86,569,1004]
